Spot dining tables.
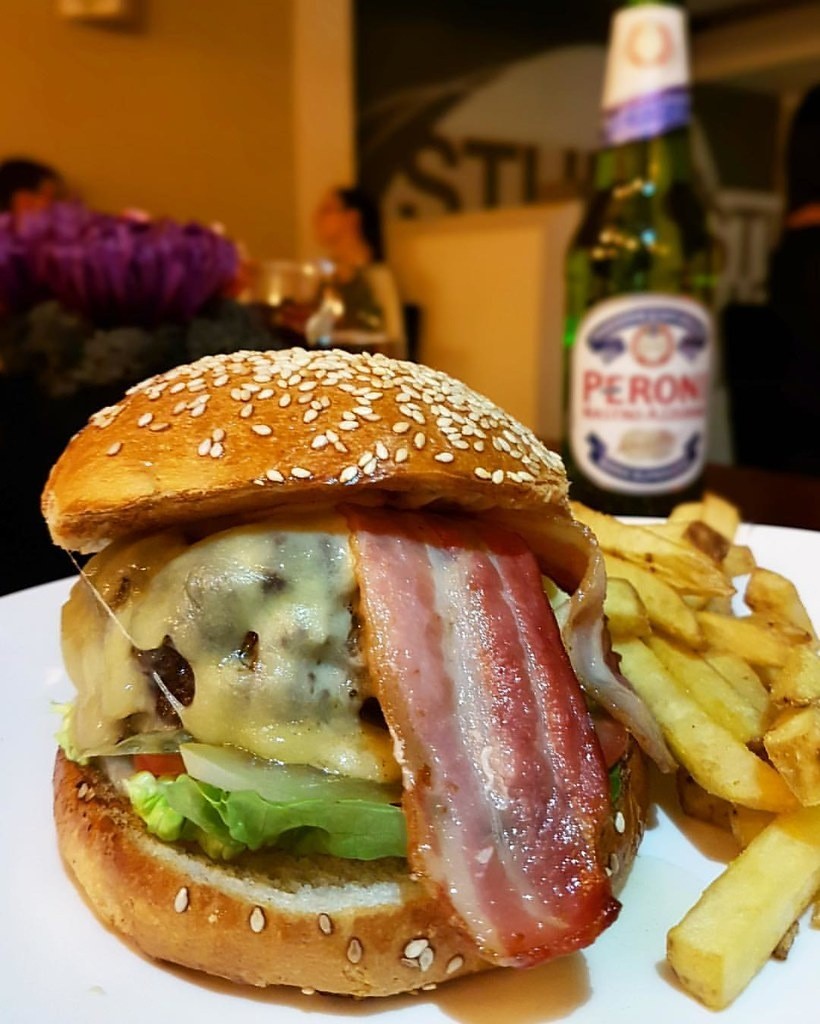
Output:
[536,438,820,540]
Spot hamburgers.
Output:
[31,347,675,1003]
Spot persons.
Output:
[0,156,63,215]
[276,187,407,358]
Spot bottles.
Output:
[561,0,720,515]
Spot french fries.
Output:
[559,494,820,1012]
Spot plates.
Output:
[0,518,820,1024]
